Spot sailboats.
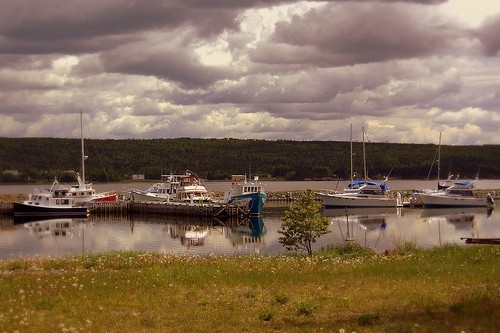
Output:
[10,106,136,220]
[313,122,500,210]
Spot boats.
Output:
[127,166,213,204]
[220,164,267,217]
[460,236,500,245]
[230,215,267,246]
